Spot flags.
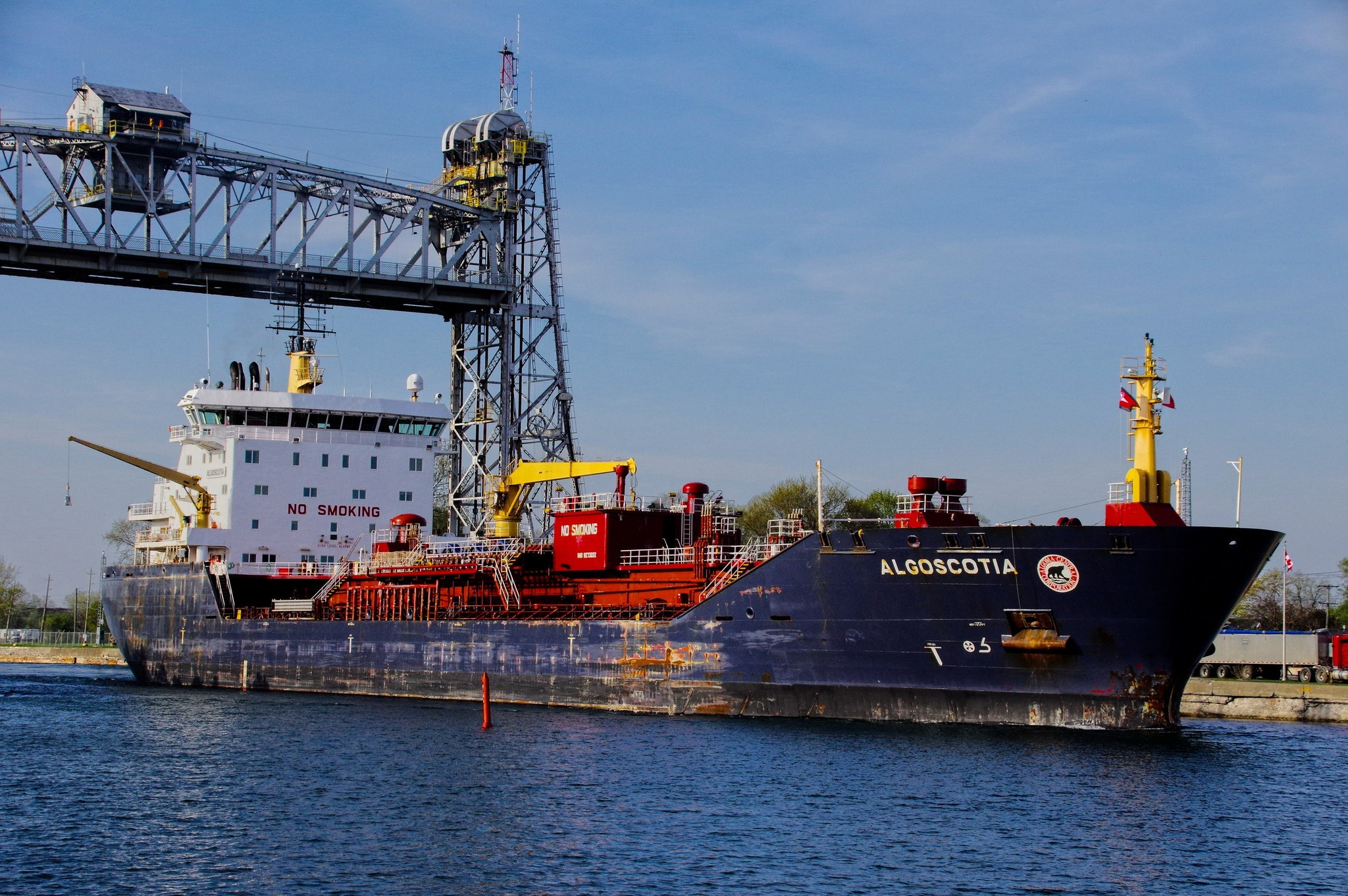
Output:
[1156,386,1175,408]
[1285,552,1293,572]
[1119,387,1141,413]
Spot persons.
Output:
[173,554,179,563]
[211,521,217,529]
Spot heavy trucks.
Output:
[1192,628,1348,685]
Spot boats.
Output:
[65,332,1287,735]
